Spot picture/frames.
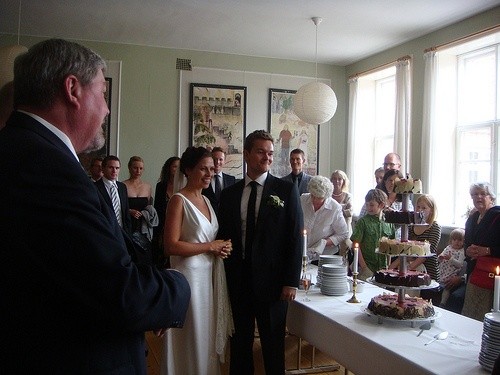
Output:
[268,88,320,178]
[190,83,247,180]
[77,77,112,181]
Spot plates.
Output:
[320,264,348,296]
[316,255,344,288]
[478,312,500,371]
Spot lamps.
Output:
[292,17,338,126]
[0,0,29,86]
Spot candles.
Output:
[494,265,500,311]
[304,230,307,256]
[354,242,358,273]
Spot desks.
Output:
[286,262,491,375]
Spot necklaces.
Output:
[333,192,342,196]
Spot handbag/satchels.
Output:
[130,216,153,258]
[470,255,500,291]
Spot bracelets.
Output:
[486,248,488,254]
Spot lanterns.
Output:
[294,83,337,125]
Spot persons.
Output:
[281,149,312,195]
[121,156,154,256]
[359,153,415,241]
[87,157,104,182]
[216,130,304,375]
[153,156,180,268]
[420,228,471,310]
[408,194,441,292]
[0,39,191,375]
[461,180,500,322]
[93,155,133,238]
[350,189,395,282]
[161,145,232,375]
[300,175,350,267]
[330,170,354,256]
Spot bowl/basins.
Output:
[350,281,365,293]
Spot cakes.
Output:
[367,173,435,320]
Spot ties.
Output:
[110,184,123,227]
[245,181,259,261]
[214,175,222,195]
[294,176,298,189]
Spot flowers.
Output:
[268,194,284,209]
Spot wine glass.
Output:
[302,273,311,302]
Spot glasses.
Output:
[473,192,490,197]
[383,163,400,166]
